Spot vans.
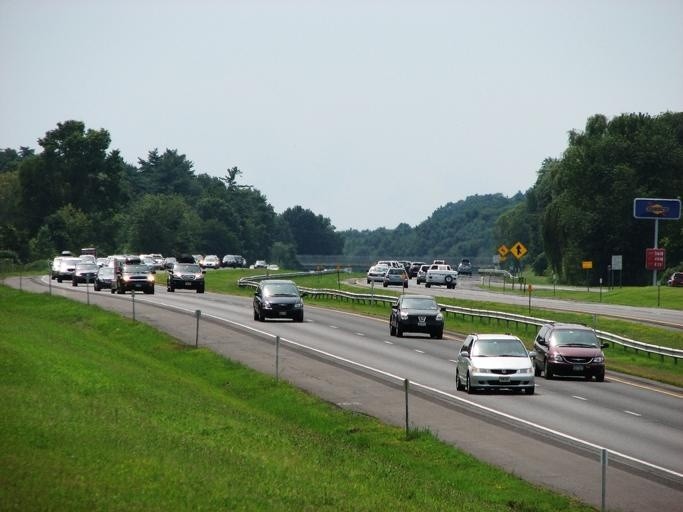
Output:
[432,259,446,265]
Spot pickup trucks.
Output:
[426,262,458,290]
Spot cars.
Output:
[407,262,428,278]
[48,247,205,295]
[666,271,683,286]
[221,254,279,270]
[368,259,412,287]
[203,254,219,269]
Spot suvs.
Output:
[532,321,609,383]
[457,258,472,276]
[452,332,539,396]
[386,294,446,342]
[415,265,431,284]
[252,279,304,322]
